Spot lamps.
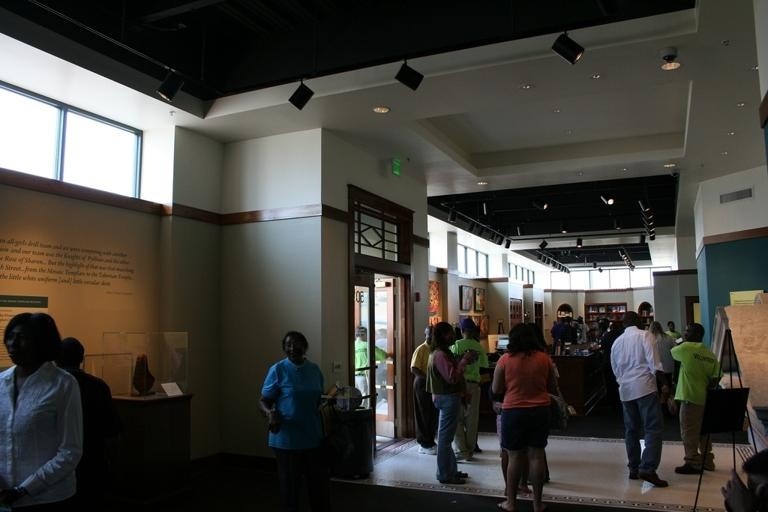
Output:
[638,199,656,240]
[551,31,584,66]
[288,80,314,110]
[155,72,184,102]
[576,238,582,248]
[601,192,614,205]
[537,251,571,273]
[539,239,548,250]
[394,58,424,92]
[560,222,568,234]
[448,203,512,249]
[614,218,622,230]
[617,247,634,271]
[533,199,548,211]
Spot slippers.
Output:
[496,502,514,512]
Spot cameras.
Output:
[466,348,472,353]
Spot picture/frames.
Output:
[475,287,486,312]
[460,285,473,311]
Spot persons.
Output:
[720,446,767,510]
[60,336,113,480]
[491,401,531,494]
[640,304,652,327]
[258,331,325,462]
[667,324,680,339]
[529,324,569,485]
[427,321,475,485]
[650,322,674,399]
[593,317,621,418]
[455,326,483,452]
[670,324,723,474]
[450,317,488,458]
[490,323,545,511]
[0,312,84,511]
[410,328,438,455]
[611,311,669,488]
[356,326,388,409]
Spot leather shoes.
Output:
[637,472,668,487]
[456,471,468,478]
[440,479,465,484]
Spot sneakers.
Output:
[473,443,482,454]
[629,469,640,481]
[418,445,438,455]
[504,488,522,497]
[453,452,474,464]
[675,464,704,474]
[521,487,532,494]
[703,462,716,472]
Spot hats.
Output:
[460,318,475,330]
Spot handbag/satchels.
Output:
[320,403,338,436]
[550,394,577,420]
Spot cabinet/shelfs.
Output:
[330,407,374,481]
[585,302,628,332]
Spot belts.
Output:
[467,380,481,384]
